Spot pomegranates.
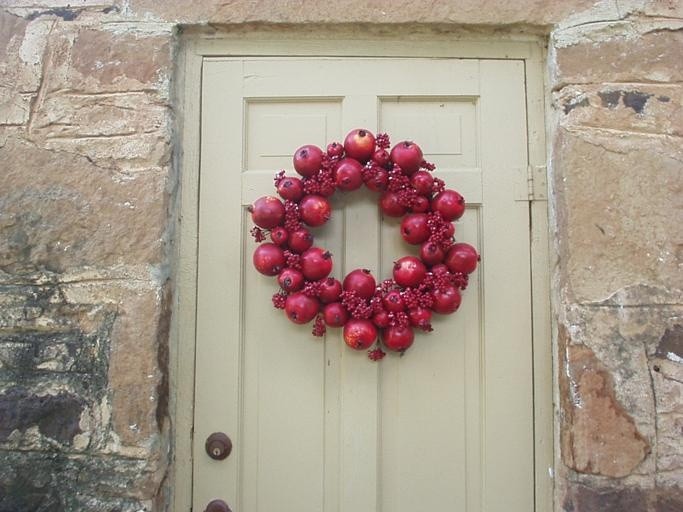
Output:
[244,129,480,362]
